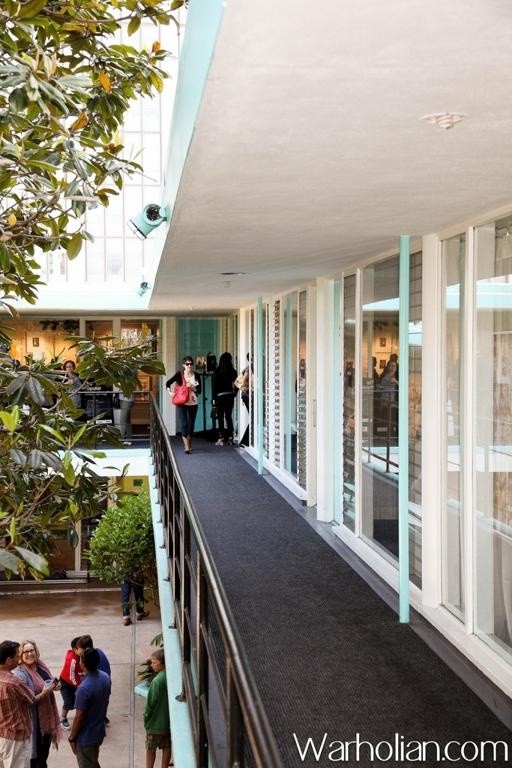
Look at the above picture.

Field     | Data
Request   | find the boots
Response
[182,435,192,453]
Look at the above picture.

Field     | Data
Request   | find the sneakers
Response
[60,717,71,731]
[137,610,149,620]
[124,618,132,626]
[215,438,245,447]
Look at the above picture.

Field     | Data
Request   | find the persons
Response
[76,634,112,725]
[59,637,87,730]
[120,539,151,626]
[16,352,253,454]
[367,353,397,443]
[68,648,113,768]
[142,648,172,768]
[1,640,37,768]
[11,638,62,768]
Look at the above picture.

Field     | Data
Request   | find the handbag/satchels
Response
[172,385,189,405]
[235,372,249,392]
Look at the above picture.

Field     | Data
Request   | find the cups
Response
[44,679,53,688]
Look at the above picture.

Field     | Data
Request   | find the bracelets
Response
[68,736,76,743]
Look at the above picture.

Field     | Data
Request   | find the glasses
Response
[186,363,193,366]
